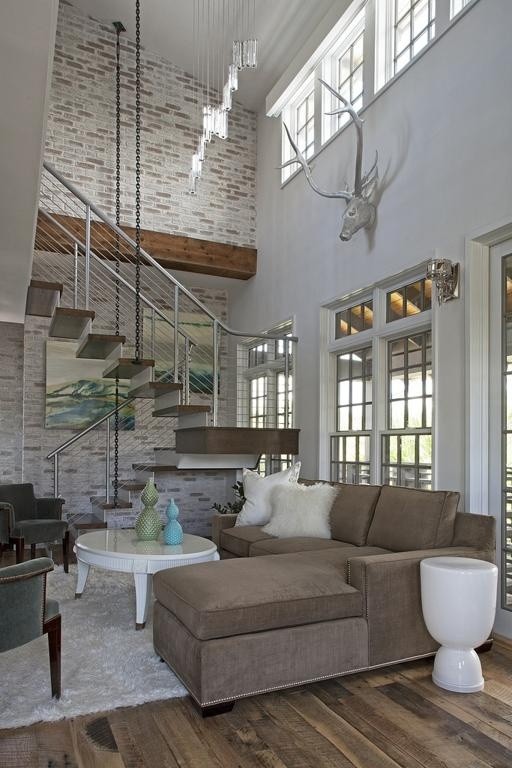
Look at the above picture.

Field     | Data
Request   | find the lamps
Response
[425,258,459,303]
[187,0,257,195]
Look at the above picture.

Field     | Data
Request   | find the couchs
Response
[151,514,498,719]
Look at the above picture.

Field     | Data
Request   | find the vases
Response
[163,498,184,545]
[134,477,163,541]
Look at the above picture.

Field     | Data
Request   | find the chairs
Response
[0,484,70,573]
[0,557,61,702]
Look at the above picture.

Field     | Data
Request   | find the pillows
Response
[233,451,460,553]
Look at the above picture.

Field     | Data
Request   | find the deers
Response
[273,77,378,241]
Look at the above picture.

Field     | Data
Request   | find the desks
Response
[418,557,497,693]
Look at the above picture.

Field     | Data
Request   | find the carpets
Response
[0,565,188,729]
